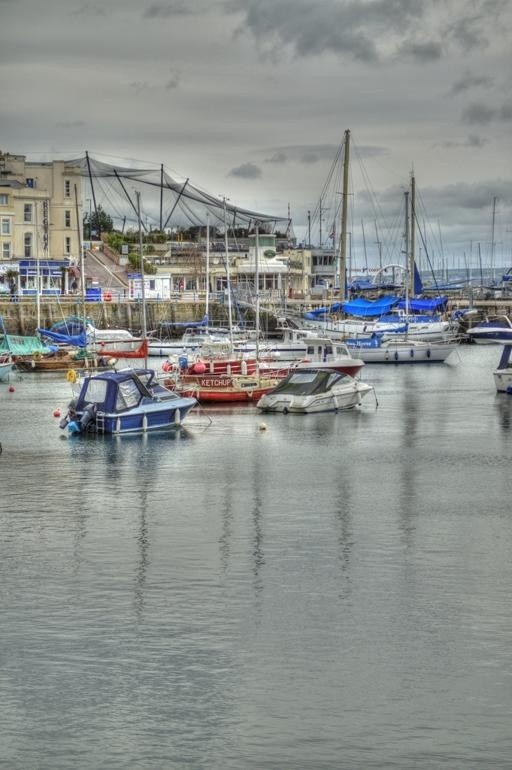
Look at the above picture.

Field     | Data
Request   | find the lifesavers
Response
[67,369,76,383]
[32,351,41,361]
[77,348,87,358]
[103,290,112,301]
